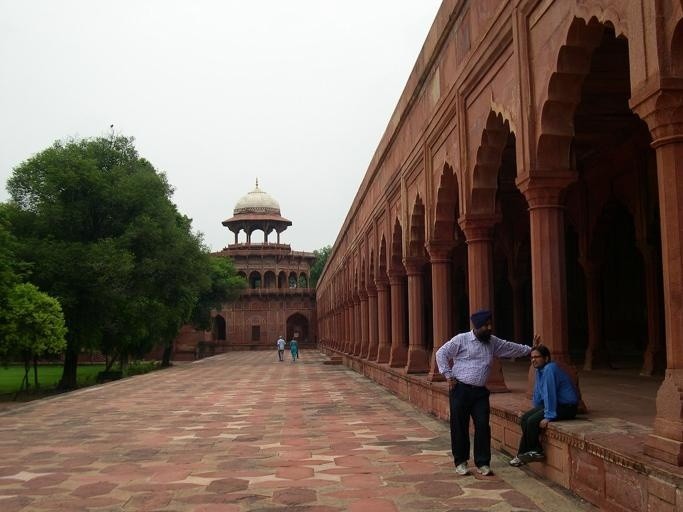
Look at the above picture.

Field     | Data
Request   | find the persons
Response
[289,336,298,361]
[508,345,583,468]
[276,335,285,361]
[434,309,543,477]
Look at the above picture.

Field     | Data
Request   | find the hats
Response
[470,310,493,329]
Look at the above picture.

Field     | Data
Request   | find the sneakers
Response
[456,460,470,475]
[509,448,545,467]
[477,464,490,475]
